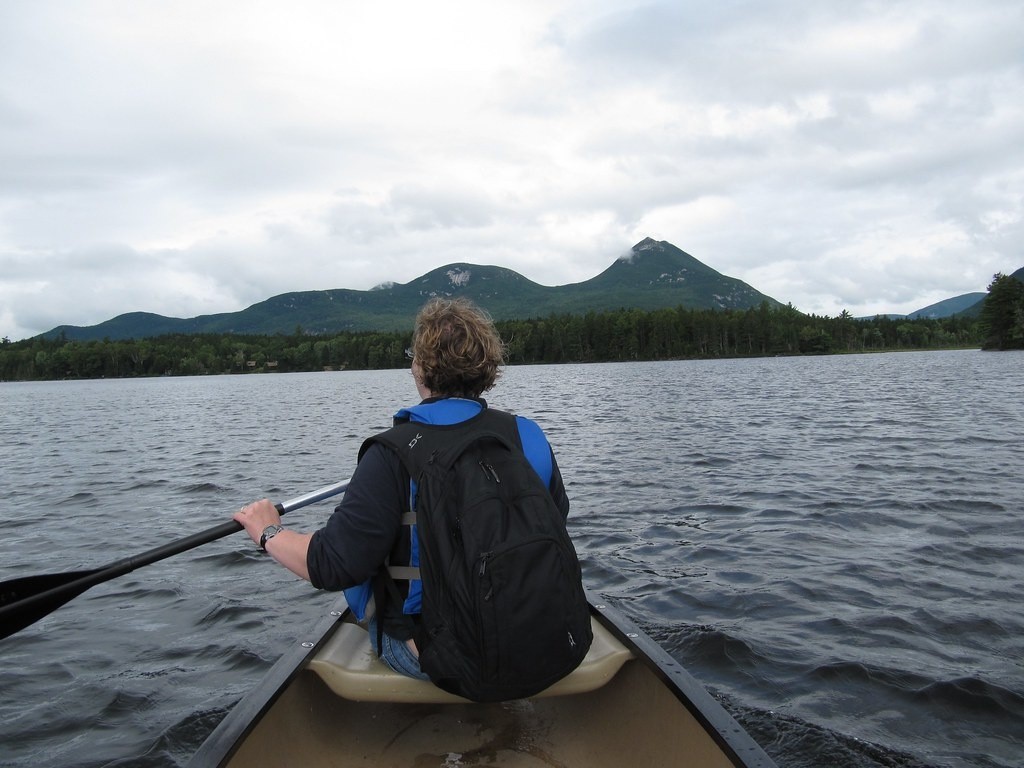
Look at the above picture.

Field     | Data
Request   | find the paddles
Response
[0,477,351,640]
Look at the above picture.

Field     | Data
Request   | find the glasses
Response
[404,346,417,359]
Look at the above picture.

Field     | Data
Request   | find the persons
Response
[232,298,571,681]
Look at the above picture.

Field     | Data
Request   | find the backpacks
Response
[362,406,596,705]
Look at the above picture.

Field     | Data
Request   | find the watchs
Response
[258,526,284,548]
